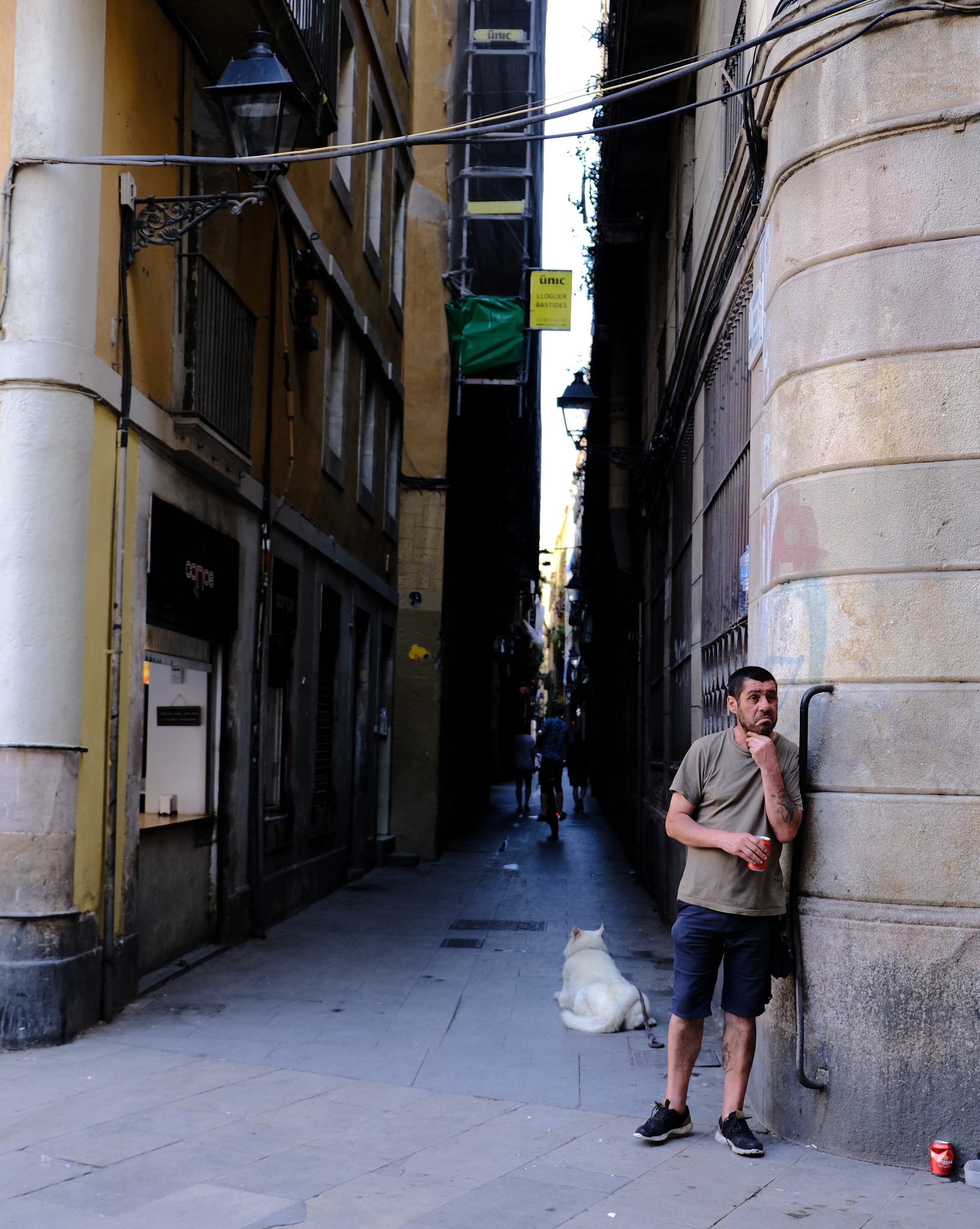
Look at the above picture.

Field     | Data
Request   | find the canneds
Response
[929,1139,953,1176]
[747,835,770,871]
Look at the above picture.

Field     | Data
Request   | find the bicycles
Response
[531,751,574,840]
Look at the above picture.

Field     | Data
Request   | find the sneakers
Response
[715,1109,764,1156]
[634,1097,693,1141]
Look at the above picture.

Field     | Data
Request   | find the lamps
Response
[111,20,310,275]
[554,370,645,470]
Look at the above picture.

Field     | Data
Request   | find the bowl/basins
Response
[963,1160,980,1187]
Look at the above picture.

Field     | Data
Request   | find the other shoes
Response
[524,805,530,812]
[559,812,567,821]
[573,799,584,811]
[537,813,548,822]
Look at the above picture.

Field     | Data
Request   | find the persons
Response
[633,665,804,1156]
[512,701,592,818]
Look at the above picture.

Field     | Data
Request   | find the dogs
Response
[553,921,656,1033]
